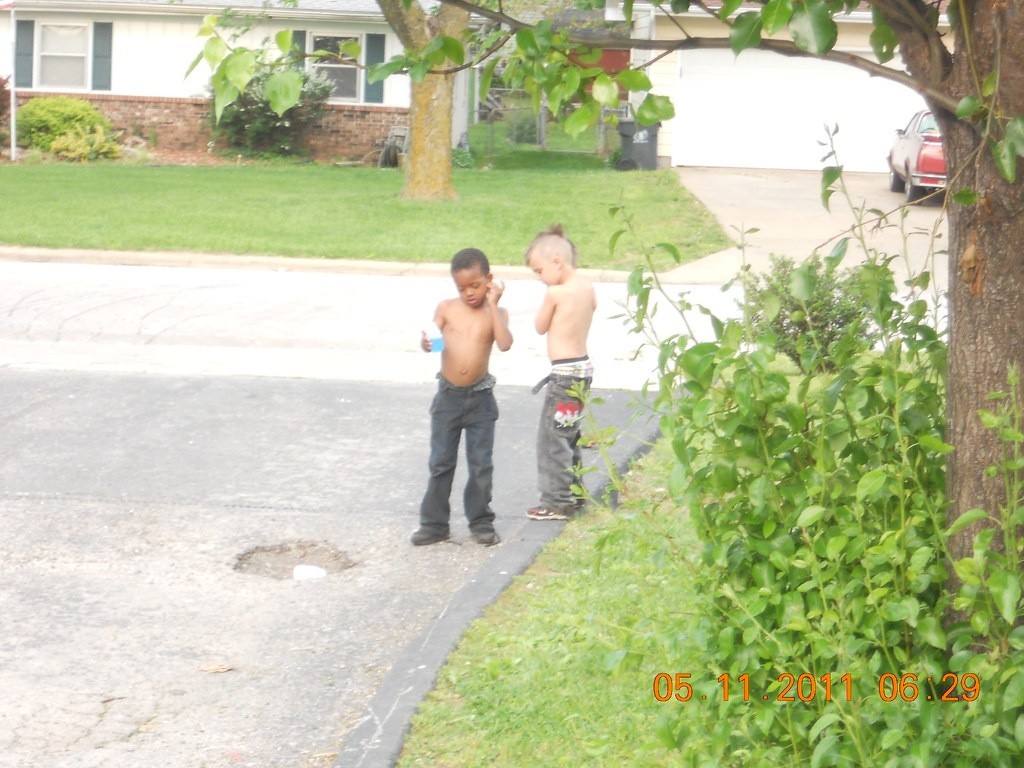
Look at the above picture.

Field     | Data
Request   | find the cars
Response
[886,109,947,203]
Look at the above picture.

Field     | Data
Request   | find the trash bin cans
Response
[616,117,662,171]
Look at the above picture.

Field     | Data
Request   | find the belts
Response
[532,376,592,395]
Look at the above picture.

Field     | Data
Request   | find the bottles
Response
[292,565,326,580]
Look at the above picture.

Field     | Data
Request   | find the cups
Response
[430,335,444,352]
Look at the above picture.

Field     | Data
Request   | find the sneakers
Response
[474,531,495,544]
[572,502,586,509]
[412,528,450,545]
[525,505,571,520]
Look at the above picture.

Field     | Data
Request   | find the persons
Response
[411,247,513,547]
[524,222,597,521]
